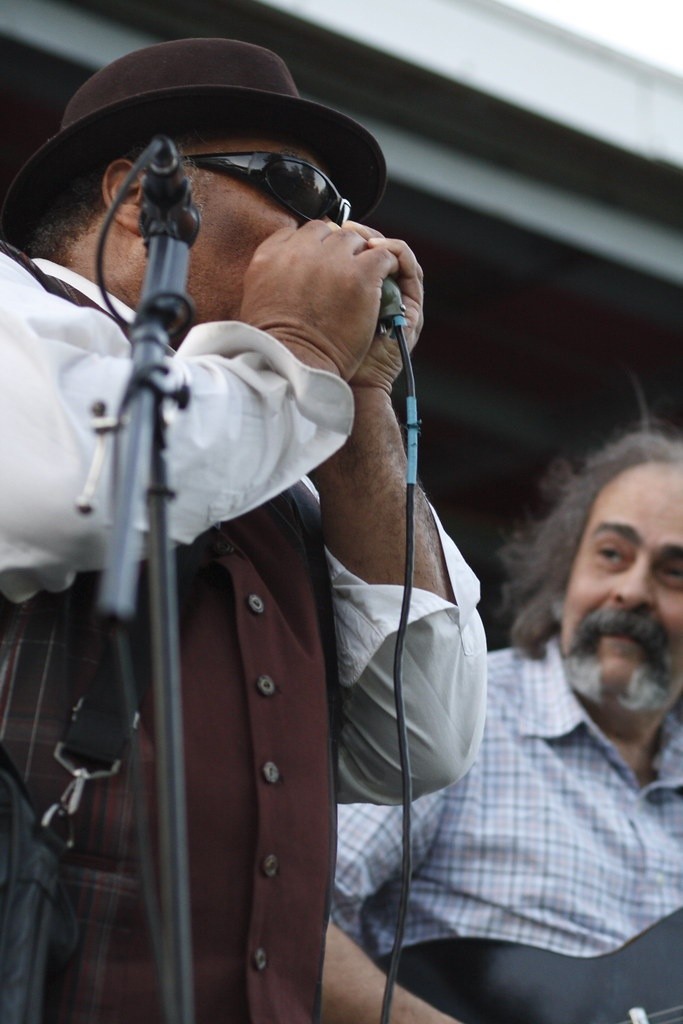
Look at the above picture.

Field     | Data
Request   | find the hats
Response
[0,36,385,240]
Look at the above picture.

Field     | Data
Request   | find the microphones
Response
[136,137,200,249]
[379,275,406,334]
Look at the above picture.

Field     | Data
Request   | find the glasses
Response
[183,150,352,233]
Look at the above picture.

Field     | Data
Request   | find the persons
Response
[0,37,491,1024]
[319,427,683,1024]
